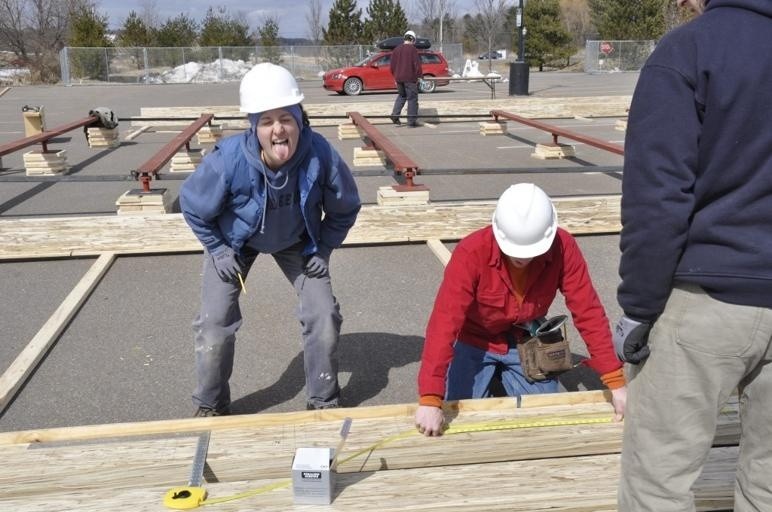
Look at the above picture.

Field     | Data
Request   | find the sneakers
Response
[390,114,401,124]
[195,407,229,417]
[306,400,343,410]
[407,123,417,127]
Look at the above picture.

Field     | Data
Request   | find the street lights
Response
[522,25,528,57]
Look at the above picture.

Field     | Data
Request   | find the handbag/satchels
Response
[517,334,574,383]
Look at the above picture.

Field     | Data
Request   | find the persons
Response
[390,30,424,127]
[614,0,772,512]
[179,61,362,418]
[414,183,628,437]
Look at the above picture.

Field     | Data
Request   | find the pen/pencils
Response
[238,273,246,294]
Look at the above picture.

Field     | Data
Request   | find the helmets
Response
[404,30,416,42]
[492,182,559,258]
[239,63,304,114]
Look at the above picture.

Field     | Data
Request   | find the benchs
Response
[421,76,502,99]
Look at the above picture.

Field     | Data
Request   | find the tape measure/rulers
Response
[187,428,211,488]
[165,416,624,507]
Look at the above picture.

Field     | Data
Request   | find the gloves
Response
[302,251,329,278]
[208,244,246,282]
[613,313,652,364]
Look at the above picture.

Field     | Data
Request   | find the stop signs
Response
[598,41,613,55]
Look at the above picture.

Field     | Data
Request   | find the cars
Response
[322,34,450,96]
[478,50,503,60]
